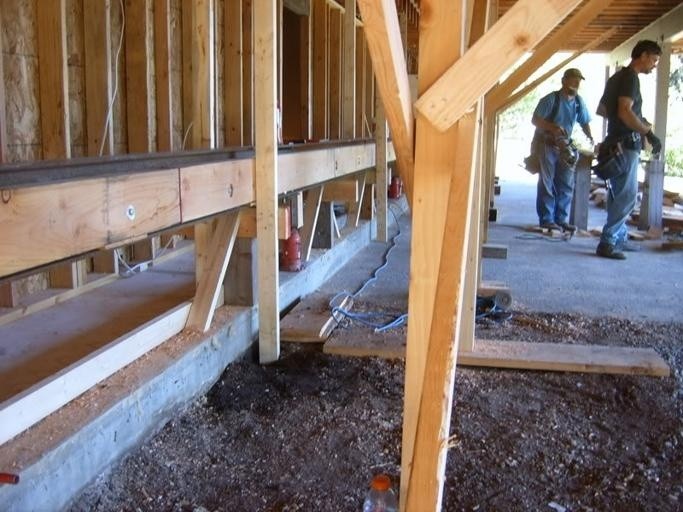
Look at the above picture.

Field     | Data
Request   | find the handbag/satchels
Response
[590,136,629,181]
[523,131,545,175]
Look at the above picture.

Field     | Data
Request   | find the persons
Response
[596,42,663,261]
[529,67,594,232]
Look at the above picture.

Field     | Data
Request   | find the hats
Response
[565,68,584,81]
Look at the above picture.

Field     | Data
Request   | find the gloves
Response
[644,128,662,155]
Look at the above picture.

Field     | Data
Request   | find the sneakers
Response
[596,242,626,259]
[557,222,578,232]
[616,240,641,252]
[540,222,561,231]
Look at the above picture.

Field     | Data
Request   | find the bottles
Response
[391,176,400,200]
[279,228,302,272]
[362,476,396,511]
